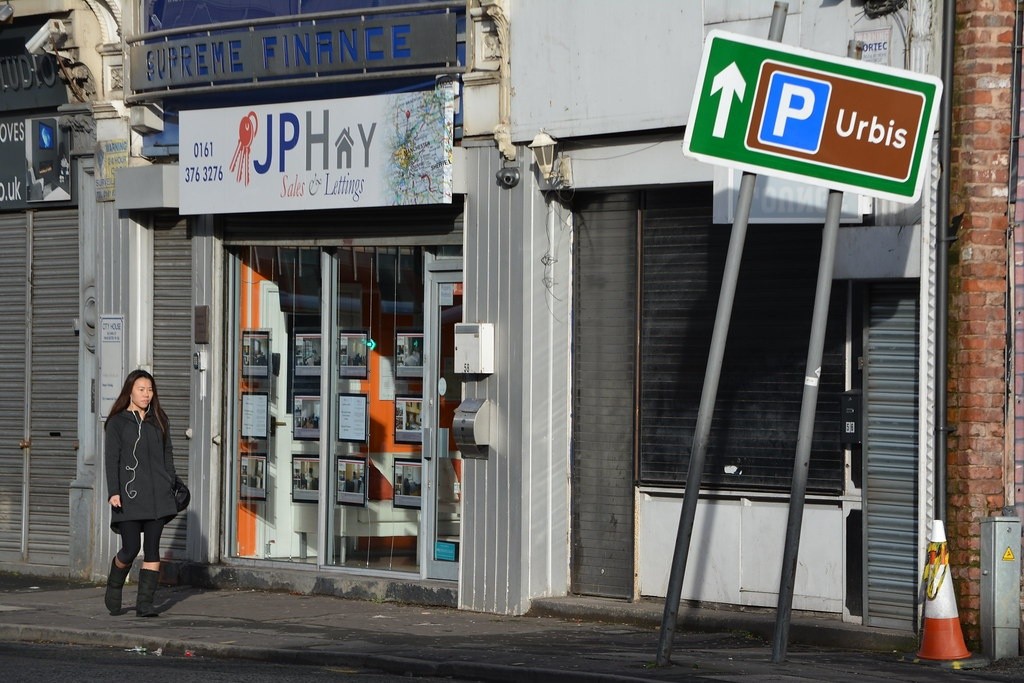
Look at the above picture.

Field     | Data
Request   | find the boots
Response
[136,568,160,617]
[105,556,132,613]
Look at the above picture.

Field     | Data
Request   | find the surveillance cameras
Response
[25,19,66,55]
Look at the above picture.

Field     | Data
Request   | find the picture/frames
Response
[336,455,367,508]
[338,392,367,444]
[241,391,268,441]
[242,331,270,379]
[393,457,422,511]
[240,453,267,501]
[291,454,319,504]
[294,331,321,377]
[394,394,422,446]
[339,330,370,380]
[395,329,424,380]
[293,392,320,441]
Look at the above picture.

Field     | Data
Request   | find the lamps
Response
[527,128,572,186]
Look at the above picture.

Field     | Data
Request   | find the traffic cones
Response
[917,542,972,659]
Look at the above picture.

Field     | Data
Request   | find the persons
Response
[103,369,178,619]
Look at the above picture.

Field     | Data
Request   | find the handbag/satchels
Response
[174,476,190,512]
